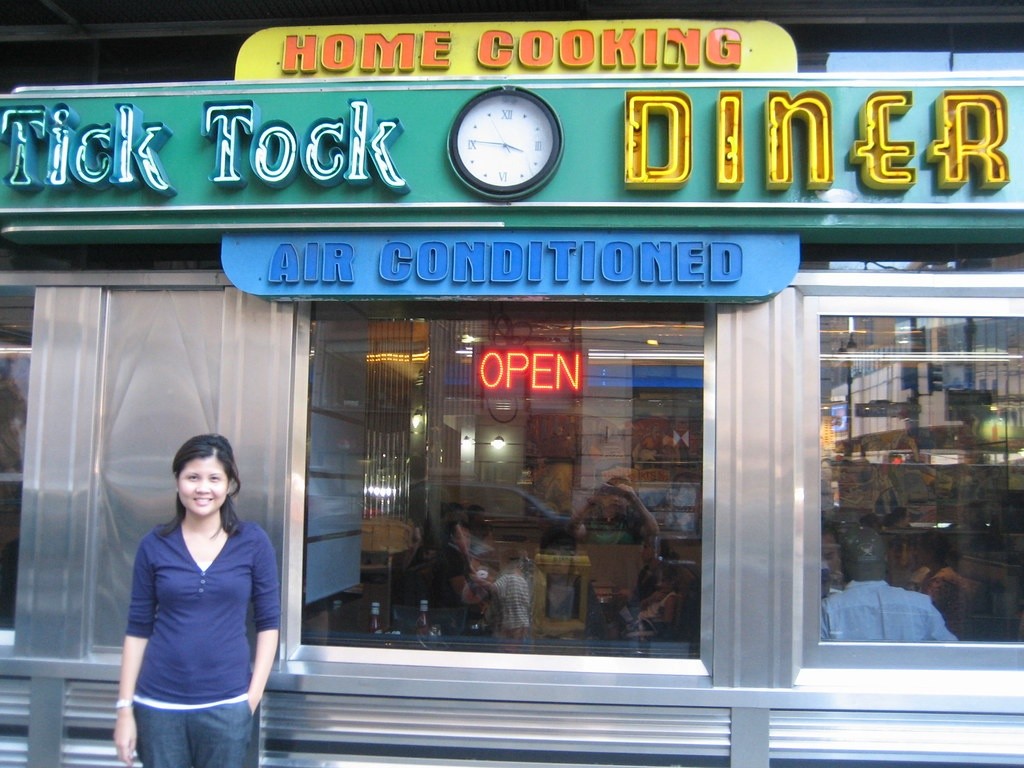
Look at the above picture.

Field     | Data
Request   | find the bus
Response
[835,424,991,504]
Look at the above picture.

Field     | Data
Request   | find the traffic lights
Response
[900,362,914,389]
[928,364,944,396]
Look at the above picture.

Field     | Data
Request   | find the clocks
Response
[445,82,567,203]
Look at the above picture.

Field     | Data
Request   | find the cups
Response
[429,624,441,636]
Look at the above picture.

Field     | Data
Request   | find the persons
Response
[913,534,1004,623]
[823,530,957,642]
[112,434,281,768]
[818,433,1013,597]
[379,455,702,649]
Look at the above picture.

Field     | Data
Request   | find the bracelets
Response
[115,699,133,709]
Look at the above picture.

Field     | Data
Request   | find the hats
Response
[540,525,576,549]
[842,527,886,581]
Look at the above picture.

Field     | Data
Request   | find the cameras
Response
[606,485,625,496]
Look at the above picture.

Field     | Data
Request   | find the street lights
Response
[836,335,859,457]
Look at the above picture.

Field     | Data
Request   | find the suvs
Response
[393,479,582,554]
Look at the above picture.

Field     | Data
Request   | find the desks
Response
[881,527,996,567]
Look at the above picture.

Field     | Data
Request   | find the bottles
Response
[367,602,383,634]
[415,600,431,636]
[328,601,345,634]
